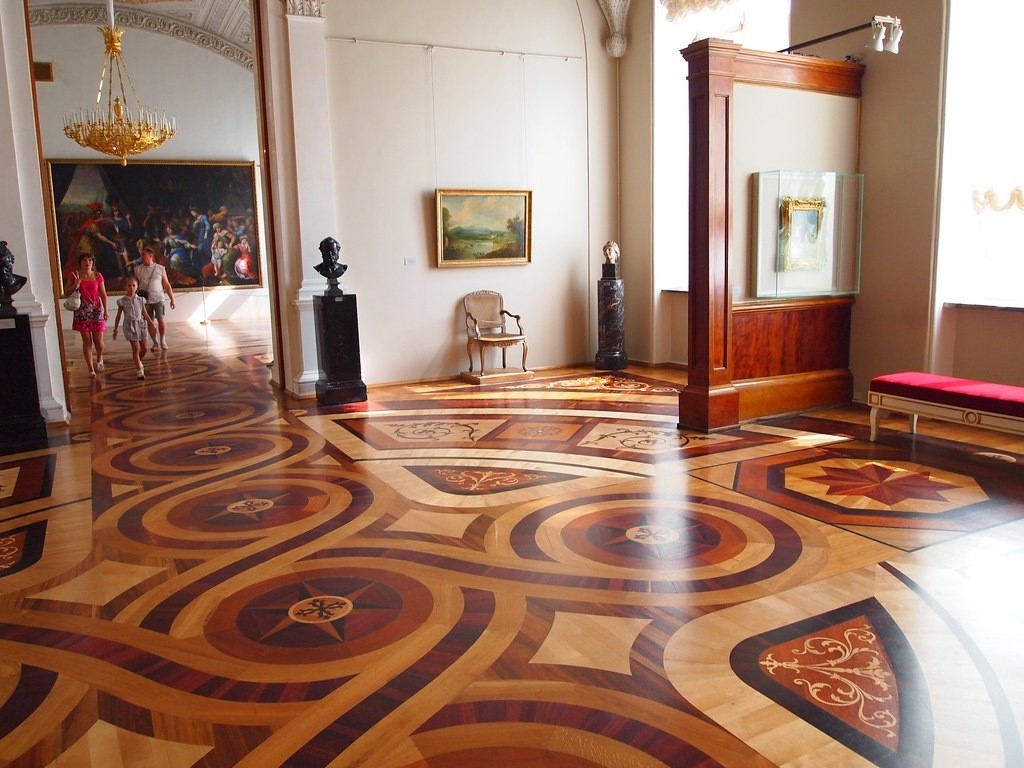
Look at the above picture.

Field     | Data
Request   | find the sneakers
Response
[136,361,145,380]
[151,341,169,352]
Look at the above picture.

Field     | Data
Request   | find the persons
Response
[313,237,348,279]
[64,252,110,378]
[132,248,175,352]
[112,278,157,381]
[602,240,621,263]
[0,241,28,296]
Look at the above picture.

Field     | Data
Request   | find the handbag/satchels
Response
[137,290,149,300]
[63,270,82,311]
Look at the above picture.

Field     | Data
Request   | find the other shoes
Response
[88,359,105,378]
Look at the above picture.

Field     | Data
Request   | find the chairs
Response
[462,289,529,374]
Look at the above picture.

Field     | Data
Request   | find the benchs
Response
[868,371,1024,444]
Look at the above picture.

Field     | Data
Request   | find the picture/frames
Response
[45,158,261,298]
[434,188,534,268]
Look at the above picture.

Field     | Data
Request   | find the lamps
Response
[63,0,178,166]
[866,14,905,53]
